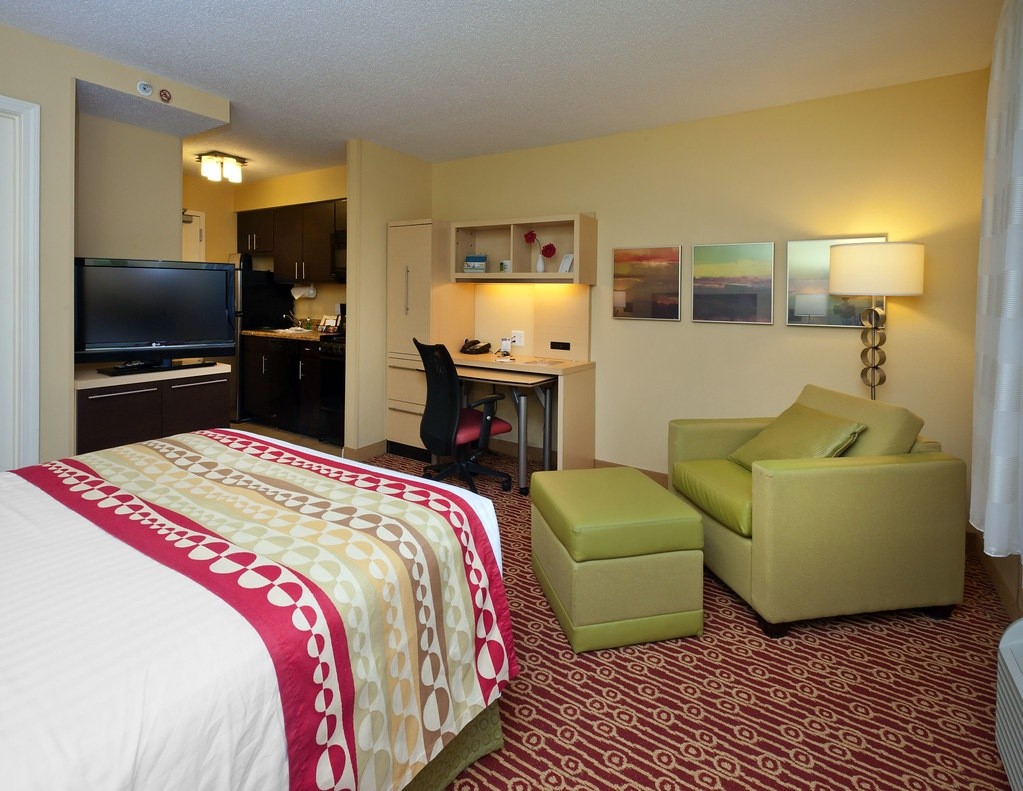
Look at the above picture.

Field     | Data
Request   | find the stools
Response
[529,466,704,653]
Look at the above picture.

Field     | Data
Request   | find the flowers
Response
[524,230,557,259]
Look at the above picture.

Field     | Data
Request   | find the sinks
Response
[263,328,313,333]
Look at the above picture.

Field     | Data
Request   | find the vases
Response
[536,254,545,273]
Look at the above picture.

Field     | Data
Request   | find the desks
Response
[416,366,557,496]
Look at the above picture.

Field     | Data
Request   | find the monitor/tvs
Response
[74,257,236,377]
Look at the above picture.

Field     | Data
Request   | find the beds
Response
[0,421,522,791]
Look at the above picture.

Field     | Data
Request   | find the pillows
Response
[726,404,866,478]
[794,382,925,461]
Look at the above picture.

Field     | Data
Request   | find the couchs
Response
[668,418,968,636]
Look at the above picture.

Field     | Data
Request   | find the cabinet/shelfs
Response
[385,214,598,472]
[76,372,264,457]
[235,198,347,448]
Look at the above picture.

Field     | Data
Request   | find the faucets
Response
[283,310,302,328]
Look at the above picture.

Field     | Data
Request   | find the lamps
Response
[196,151,248,186]
[826,241,926,402]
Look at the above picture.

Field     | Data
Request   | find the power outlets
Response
[510,330,524,347]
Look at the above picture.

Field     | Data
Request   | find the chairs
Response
[411,338,513,497]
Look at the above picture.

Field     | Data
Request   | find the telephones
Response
[459,339,491,355]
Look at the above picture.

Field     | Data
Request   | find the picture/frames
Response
[785,237,886,331]
[558,253,574,272]
[611,246,682,322]
[690,240,776,326]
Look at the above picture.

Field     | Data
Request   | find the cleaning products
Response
[306,316,311,329]
